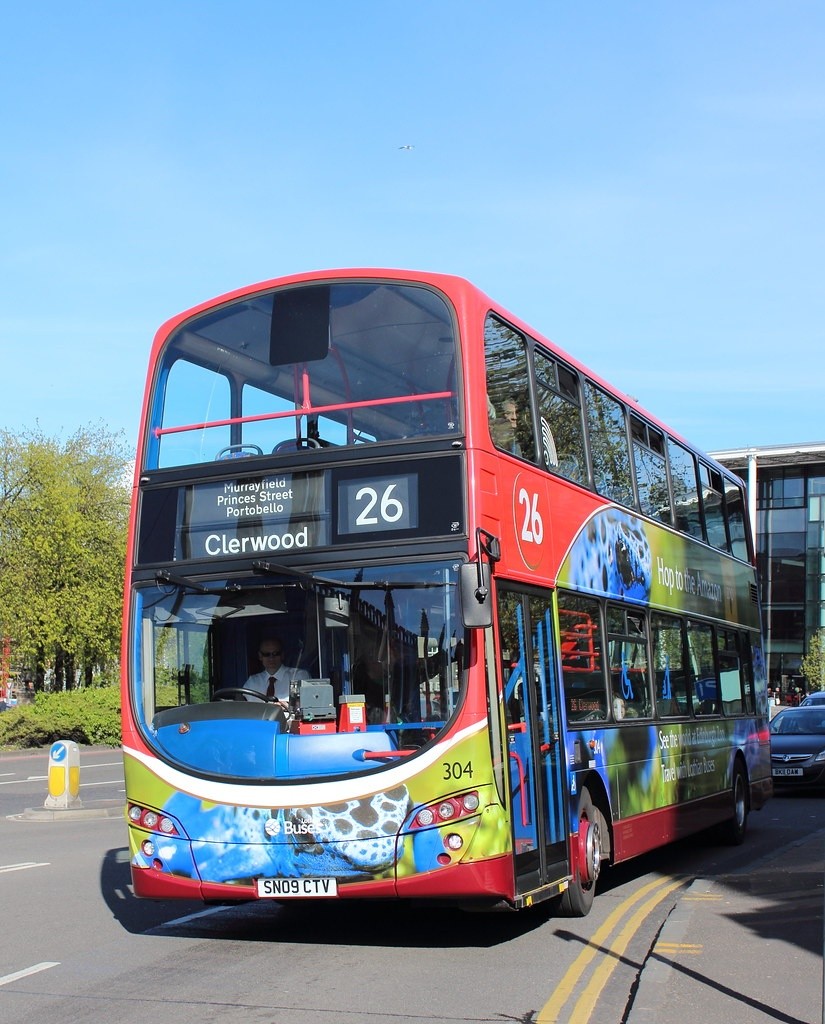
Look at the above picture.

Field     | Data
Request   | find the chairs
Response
[218,438,322,462]
[558,456,632,507]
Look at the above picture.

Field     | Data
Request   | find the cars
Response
[768,704,825,793]
[794,692,825,716]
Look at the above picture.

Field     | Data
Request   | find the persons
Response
[486,393,496,420]
[241,638,313,733]
[501,396,536,463]
[789,679,799,707]
[507,631,680,843]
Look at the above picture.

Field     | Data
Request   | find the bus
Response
[117,265,776,920]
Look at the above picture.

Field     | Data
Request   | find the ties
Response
[266,677,276,698]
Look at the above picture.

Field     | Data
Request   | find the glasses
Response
[259,649,282,657]
